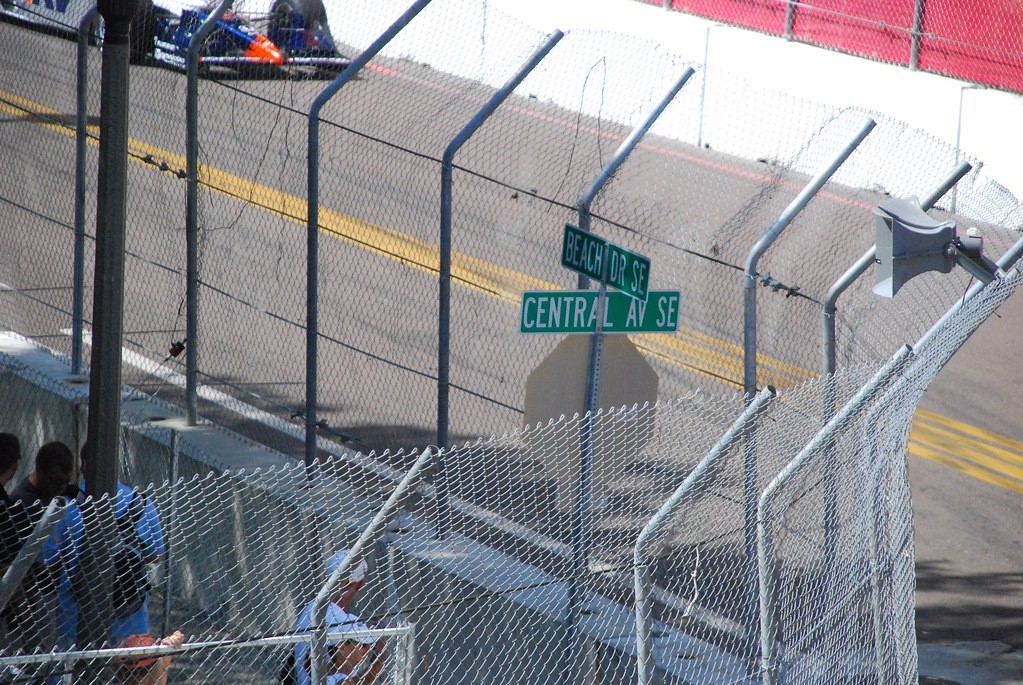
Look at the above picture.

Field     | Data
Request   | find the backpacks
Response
[76,488,148,619]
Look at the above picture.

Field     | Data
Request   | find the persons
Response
[294,549,390,685]
[0,432,184,685]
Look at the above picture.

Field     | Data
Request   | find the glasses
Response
[40,463,74,487]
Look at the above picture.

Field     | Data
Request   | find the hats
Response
[117,633,159,669]
[326,549,368,587]
[327,614,378,647]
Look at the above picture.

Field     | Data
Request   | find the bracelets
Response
[371,652,386,666]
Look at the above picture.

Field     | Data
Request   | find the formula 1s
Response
[0,0,356,76]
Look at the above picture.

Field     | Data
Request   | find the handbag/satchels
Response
[278,643,297,685]
